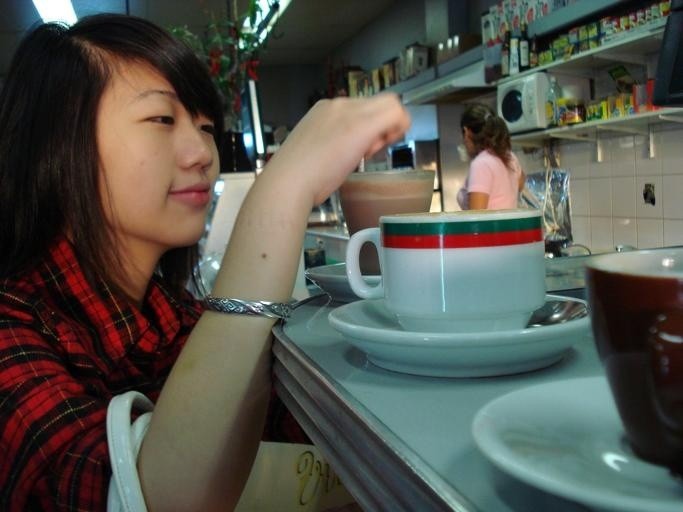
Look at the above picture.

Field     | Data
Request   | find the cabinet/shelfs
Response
[499,25,683,162]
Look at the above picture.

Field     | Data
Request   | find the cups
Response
[344,210,550,334]
[337,169,436,276]
[582,246,683,470]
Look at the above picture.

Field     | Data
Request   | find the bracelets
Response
[201,296,289,322]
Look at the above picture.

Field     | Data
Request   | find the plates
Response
[327,294,592,380]
[468,376,682,512]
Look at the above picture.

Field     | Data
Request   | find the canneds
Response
[565,98,585,125]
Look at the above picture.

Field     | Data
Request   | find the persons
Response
[455,103,525,211]
[0,13,412,511]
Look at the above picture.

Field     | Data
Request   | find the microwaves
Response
[492,71,593,139]
